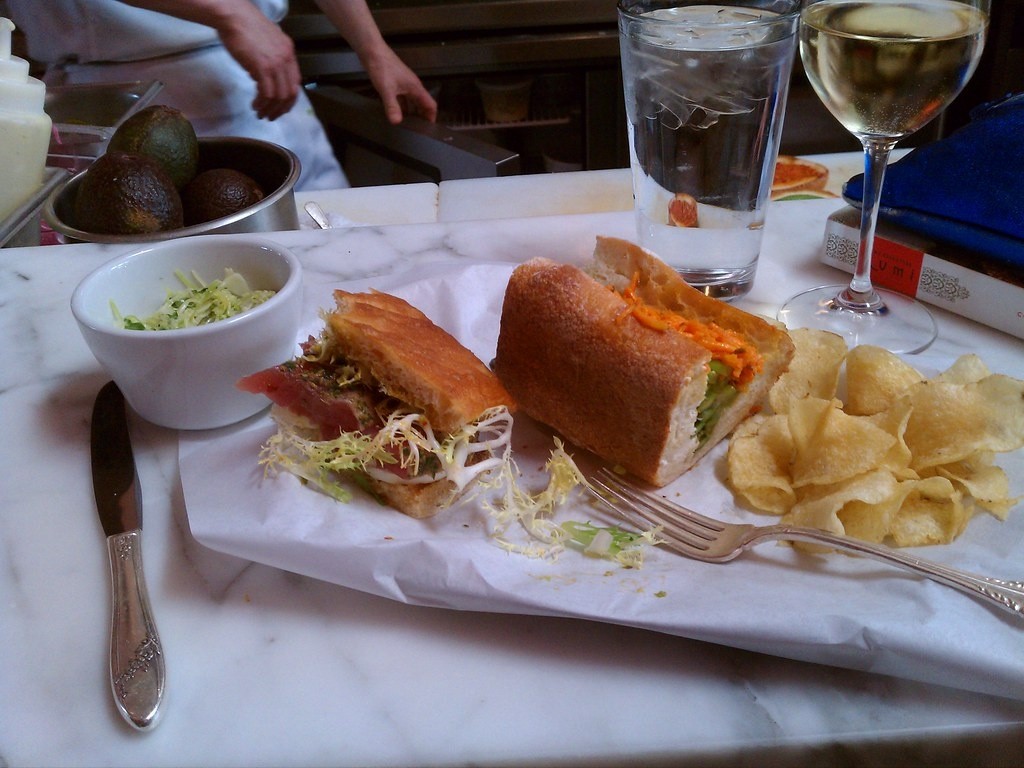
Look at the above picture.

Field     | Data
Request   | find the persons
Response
[8,0,436,193]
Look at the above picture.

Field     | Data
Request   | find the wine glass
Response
[776,0,992,356]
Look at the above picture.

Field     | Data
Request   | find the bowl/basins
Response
[474,73,533,123]
[42,136,302,243]
[70,234,304,431]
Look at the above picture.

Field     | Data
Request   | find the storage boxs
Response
[820,204,1024,340]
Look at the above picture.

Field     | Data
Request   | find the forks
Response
[585,466,1024,620]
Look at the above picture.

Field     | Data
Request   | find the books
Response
[821,206,1024,343]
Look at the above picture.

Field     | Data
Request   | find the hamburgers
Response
[235,286,669,571]
[491,234,795,486]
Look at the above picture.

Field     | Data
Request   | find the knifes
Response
[89,380,168,731]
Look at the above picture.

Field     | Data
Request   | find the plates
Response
[768,154,841,201]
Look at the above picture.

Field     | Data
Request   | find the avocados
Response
[71,104,265,235]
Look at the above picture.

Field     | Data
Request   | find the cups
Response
[617,0,803,302]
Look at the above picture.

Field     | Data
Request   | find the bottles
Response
[0,18,53,225]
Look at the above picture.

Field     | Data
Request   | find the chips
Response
[728,327,1024,558]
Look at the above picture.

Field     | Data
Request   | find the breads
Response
[773,154,841,200]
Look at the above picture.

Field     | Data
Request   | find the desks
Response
[0,194,1024,768]
